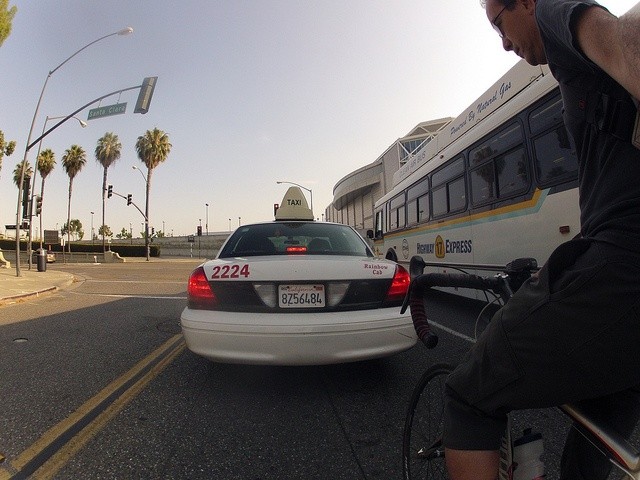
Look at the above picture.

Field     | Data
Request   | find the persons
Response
[445,0,640,480]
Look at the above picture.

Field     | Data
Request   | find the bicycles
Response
[400,258,640,479]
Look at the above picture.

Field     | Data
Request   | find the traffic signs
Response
[87,102,126,120]
[188,235,194,242]
[197,226,202,236]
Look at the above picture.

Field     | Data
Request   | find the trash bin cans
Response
[36,248,47,271]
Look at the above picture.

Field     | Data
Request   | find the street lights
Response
[277,181,312,209]
[239,217,240,226]
[199,219,201,226]
[17,27,132,275]
[91,211,94,239]
[206,203,208,235]
[132,166,146,182]
[229,219,231,231]
[141,223,144,232]
[29,116,87,268]
[163,221,164,234]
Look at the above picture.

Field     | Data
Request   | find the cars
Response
[28,249,55,263]
[181,186,418,365]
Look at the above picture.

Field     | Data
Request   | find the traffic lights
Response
[23,178,31,206]
[108,185,112,198]
[35,194,42,217]
[152,227,154,234]
[127,194,132,205]
[134,77,158,114]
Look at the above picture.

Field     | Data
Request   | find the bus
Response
[367,59,581,306]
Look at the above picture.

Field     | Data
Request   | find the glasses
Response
[491,6,508,37]
